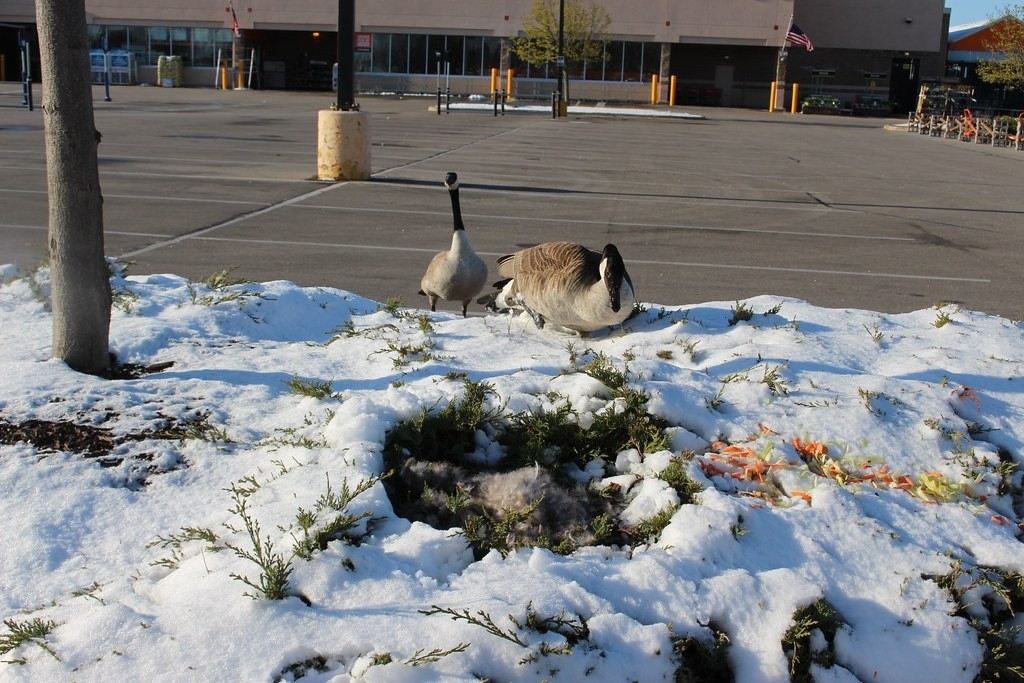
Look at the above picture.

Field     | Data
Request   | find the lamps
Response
[904,17,913,23]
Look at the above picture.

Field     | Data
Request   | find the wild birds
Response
[495,241,635,337]
[418,171,488,318]
[476,277,543,330]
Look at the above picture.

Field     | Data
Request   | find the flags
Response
[786,24,814,51]
